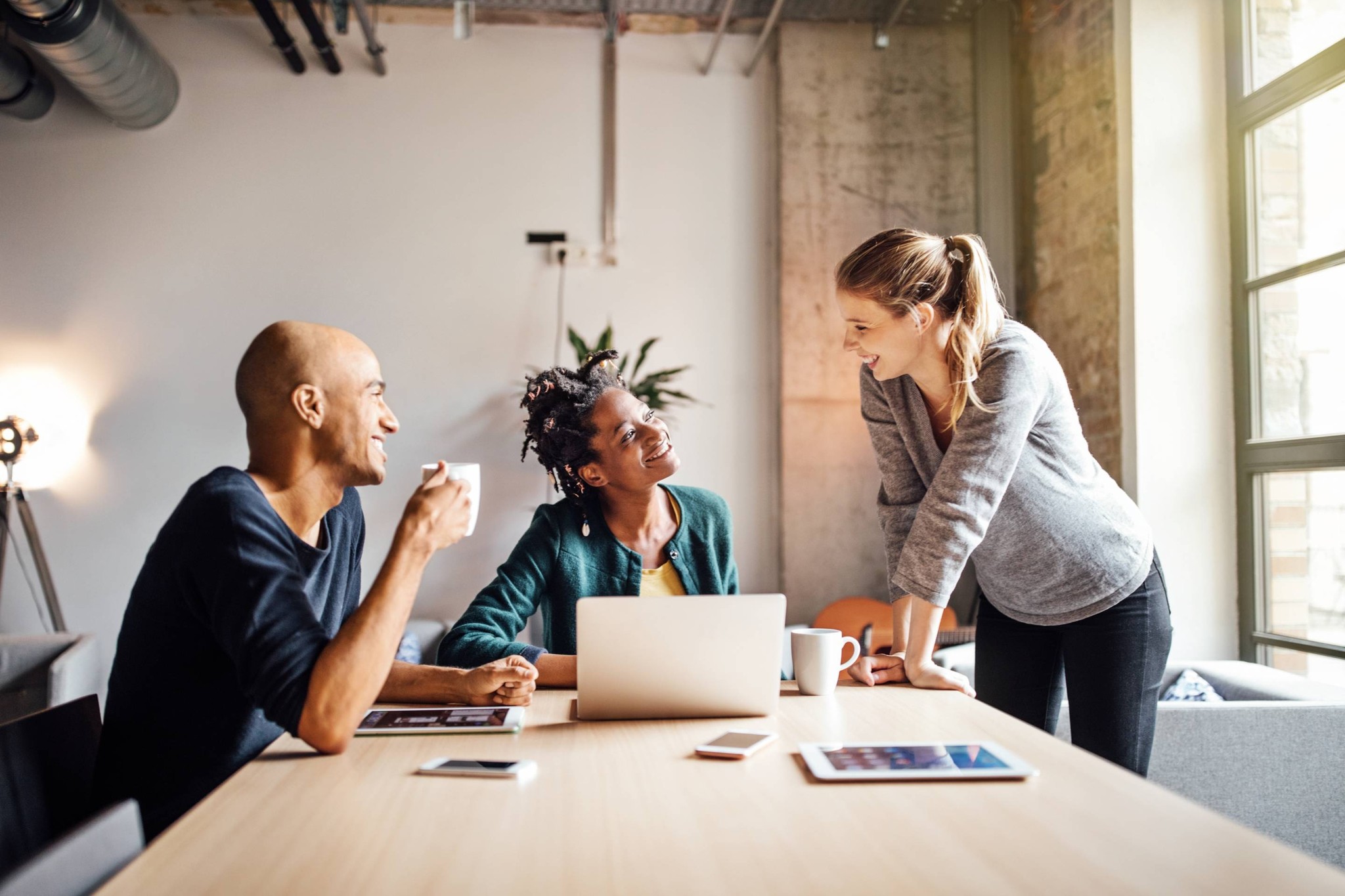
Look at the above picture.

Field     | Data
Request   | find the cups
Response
[423,463,480,537]
[791,628,861,695]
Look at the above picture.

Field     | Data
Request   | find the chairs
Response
[0,632,145,896]
[935,641,1344,870]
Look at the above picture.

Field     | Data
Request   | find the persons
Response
[435,348,740,686]
[834,227,1174,779]
[89,320,539,843]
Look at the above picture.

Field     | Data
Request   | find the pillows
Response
[1160,667,1223,702]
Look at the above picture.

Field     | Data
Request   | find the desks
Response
[88,679,1345,896]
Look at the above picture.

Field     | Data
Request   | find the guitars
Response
[812,597,976,680]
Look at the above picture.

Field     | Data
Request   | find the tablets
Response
[353,705,525,735]
[798,741,1040,781]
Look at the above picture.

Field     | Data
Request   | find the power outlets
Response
[550,240,572,264]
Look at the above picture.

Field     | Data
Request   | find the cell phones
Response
[695,730,778,759]
[418,758,534,777]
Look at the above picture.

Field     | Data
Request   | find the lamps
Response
[0,414,67,632]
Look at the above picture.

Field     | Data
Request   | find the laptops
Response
[576,594,787,719]
